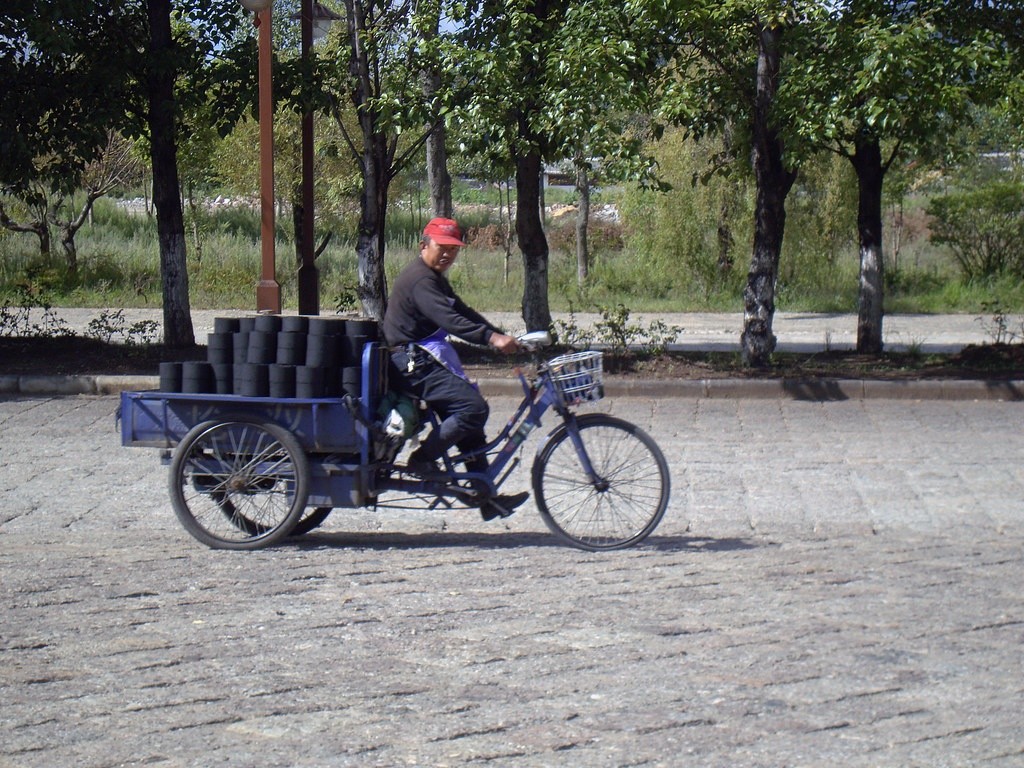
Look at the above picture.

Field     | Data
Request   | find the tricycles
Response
[115,329,672,551]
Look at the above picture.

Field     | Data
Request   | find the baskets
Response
[547,350,604,405]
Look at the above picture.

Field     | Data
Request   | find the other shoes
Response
[408,454,454,480]
[481,491,529,520]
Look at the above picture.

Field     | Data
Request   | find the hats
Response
[424,217,465,246]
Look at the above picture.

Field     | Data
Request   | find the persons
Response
[382,218,531,522]
